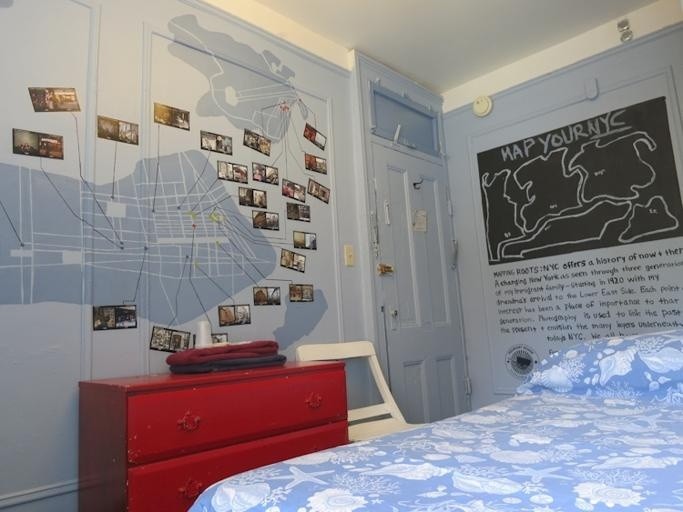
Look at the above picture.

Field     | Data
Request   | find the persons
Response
[253,166,261,180]
[44,89,58,110]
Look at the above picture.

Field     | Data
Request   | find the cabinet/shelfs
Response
[78,361,349,512]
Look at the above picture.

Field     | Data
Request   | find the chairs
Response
[294,340,431,443]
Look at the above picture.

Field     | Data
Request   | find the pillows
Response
[517,329,683,398]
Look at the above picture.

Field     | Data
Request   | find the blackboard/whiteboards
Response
[477,96,683,265]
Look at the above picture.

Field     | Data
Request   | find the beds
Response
[184,396,683,512]
